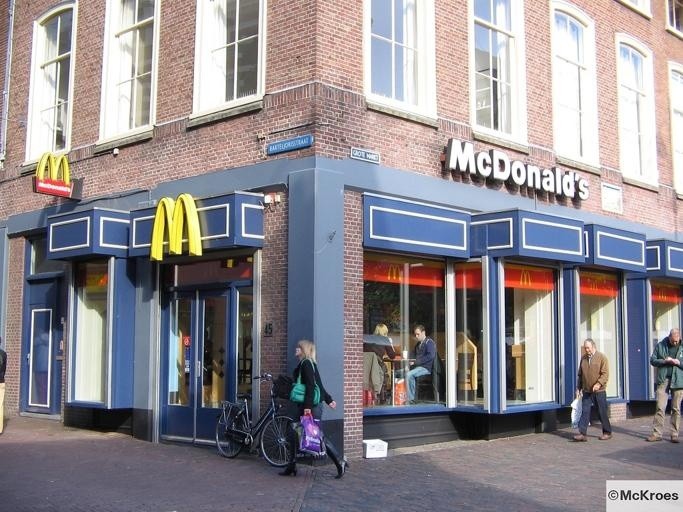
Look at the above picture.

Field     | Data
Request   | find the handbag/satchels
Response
[570,395,591,429]
[297,413,326,455]
[289,383,321,406]
[274,375,292,400]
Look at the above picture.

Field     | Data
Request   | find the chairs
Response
[416,350,446,403]
[363,351,388,408]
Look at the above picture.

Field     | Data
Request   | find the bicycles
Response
[215,372,295,468]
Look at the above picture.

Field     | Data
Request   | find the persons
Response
[571,337,614,442]
[0,336,9,435]
[363,323,397,406]
[392,323,438,403]
[645,327,682,445]
[277,340,350,480]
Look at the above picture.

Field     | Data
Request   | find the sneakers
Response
[599,433,612,440]
[671,435,680,443]
[573,434,587,442]
[647,434,662,442]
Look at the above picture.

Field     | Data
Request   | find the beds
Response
[383,359,416,406]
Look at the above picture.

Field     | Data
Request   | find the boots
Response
[278,434,298,476]
[322,435,349,479]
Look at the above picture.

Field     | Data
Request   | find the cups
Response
[402,351,408,359]
